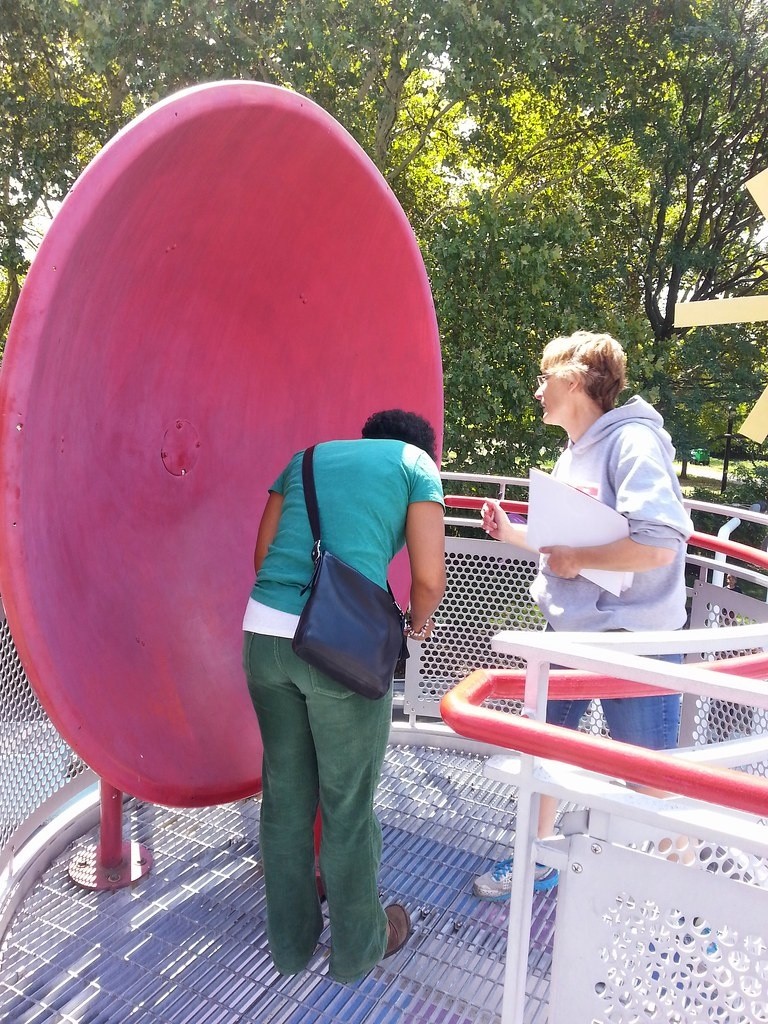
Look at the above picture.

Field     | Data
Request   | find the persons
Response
[242,409,447,983]
[723,574,743,627]
[473,332,719,955]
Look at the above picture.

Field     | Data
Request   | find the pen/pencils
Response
[485,491,503,540]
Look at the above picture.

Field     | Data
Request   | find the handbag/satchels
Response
[292,550,410,700]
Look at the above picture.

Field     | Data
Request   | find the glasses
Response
[536,372,556,386]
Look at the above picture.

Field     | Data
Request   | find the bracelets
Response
[403,603,428,638]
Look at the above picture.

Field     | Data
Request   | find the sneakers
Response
[473,857,559,900]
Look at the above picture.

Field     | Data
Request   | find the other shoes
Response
[362,904,411,961]
